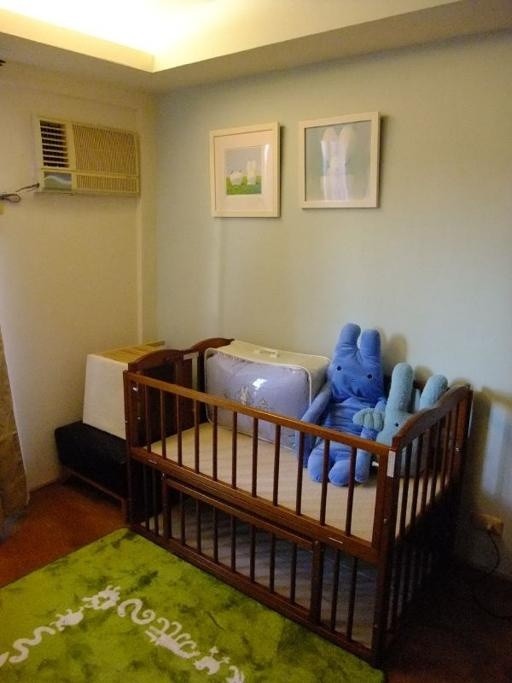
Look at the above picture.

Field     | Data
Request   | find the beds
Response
[121,337,473,669]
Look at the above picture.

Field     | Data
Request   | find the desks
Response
[54,421,169,524]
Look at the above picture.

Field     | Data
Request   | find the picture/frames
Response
[297,111,380,209]
[209,121,280,219]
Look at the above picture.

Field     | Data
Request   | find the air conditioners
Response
[31,113,140,199]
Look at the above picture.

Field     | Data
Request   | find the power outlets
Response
[474,511,504,537]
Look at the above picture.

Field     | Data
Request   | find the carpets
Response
[0,528,382,683]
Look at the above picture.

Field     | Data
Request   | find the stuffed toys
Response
[300,322,447,487]
